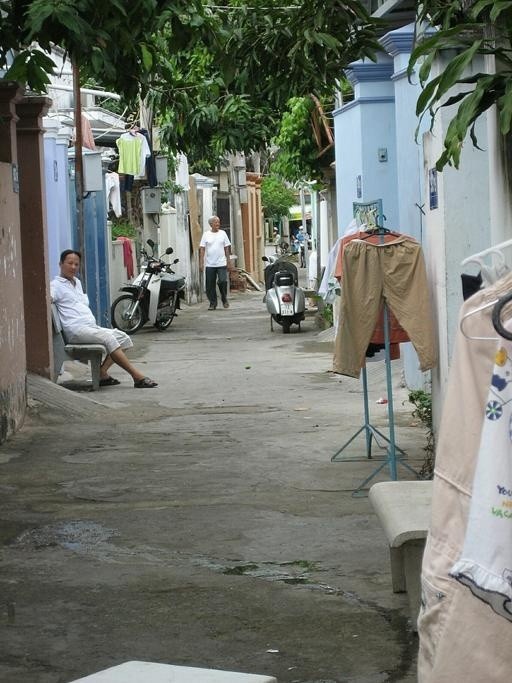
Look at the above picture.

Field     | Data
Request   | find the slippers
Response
[100,376,121,386]
[132,376,160,388]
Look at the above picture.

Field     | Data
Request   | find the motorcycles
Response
[111,236,186,333]
[257,249,307,333]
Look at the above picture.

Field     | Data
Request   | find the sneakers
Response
[207,301,229,310]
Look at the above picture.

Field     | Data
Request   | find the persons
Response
[48,249,158,387]
[198,215,234,311]
[273,226,281,256]
[291,223,301,245]
[296,225,309,268]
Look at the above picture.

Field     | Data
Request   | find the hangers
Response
[353,201,399,243]
[461,230,510,344]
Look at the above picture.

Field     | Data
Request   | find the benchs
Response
[51,303,106,390]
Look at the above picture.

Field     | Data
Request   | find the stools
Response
[369,477,435,635]
